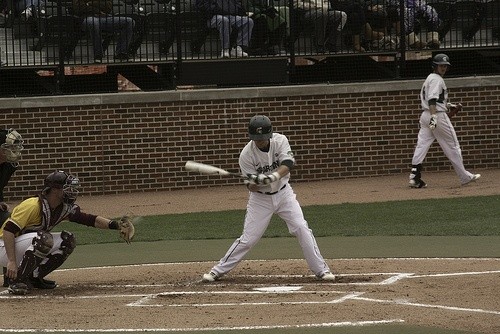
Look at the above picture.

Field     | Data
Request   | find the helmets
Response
[44,170,74,189]
[0,129,21,144]
[248,115,274,141]
[432,54,451,66]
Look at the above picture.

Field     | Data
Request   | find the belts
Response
[257,184,286,195]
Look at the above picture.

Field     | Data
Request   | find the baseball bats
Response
[447,103,463,118]
[185,160,255,181]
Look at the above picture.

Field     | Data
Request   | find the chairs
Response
[0,0,304,48]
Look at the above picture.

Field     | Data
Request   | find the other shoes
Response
[409,179,427,188]
[316,271,335,281]
[461,173,480,186]
[203,272,218,282]
[221,48,229,58]
[3,267,57,289]
[230,46,248,58]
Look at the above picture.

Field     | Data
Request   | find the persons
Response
[0,128,24,286]
[0,0,487,62]
[408,54,481,188]
[202,115,335,283]
[0,171,135,294]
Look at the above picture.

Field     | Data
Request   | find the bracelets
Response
[272,172,281,181]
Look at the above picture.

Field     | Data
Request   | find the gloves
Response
[246,172,281,193]
[447,103,462,114]
[428,115,437,128]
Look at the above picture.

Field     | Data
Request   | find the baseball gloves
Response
[118,218,136,243]
